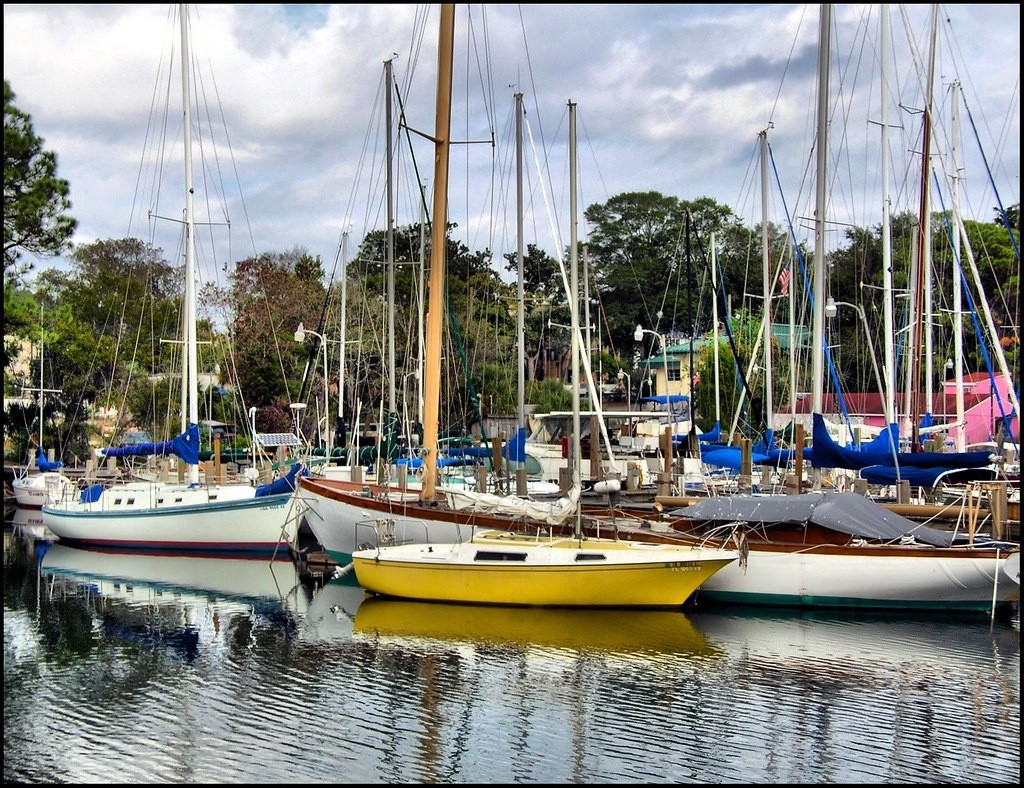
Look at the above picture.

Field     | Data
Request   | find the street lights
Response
[825,297,900,504]
[293,324,330,468]
[401,370,421,434]
[248,407,256,472]
[633,325,672,427]
[639,378,652,402]
[615,366,632,414]
[289,403,306,458]
[943,357,954,442]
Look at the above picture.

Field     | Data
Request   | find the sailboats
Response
[2,1,1021,618]
